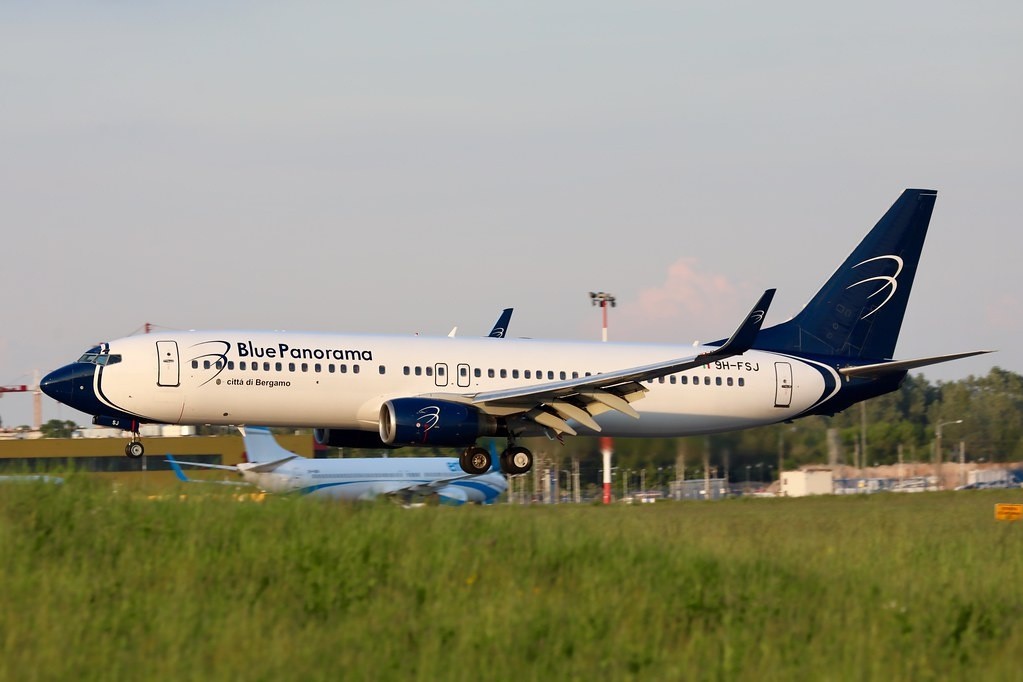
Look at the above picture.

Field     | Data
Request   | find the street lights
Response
[935,420,964,479]
[586,288,617,506]
[778,428,796,472]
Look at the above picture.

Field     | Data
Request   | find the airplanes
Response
[161,425,510,510]
[37,186,997,478]
